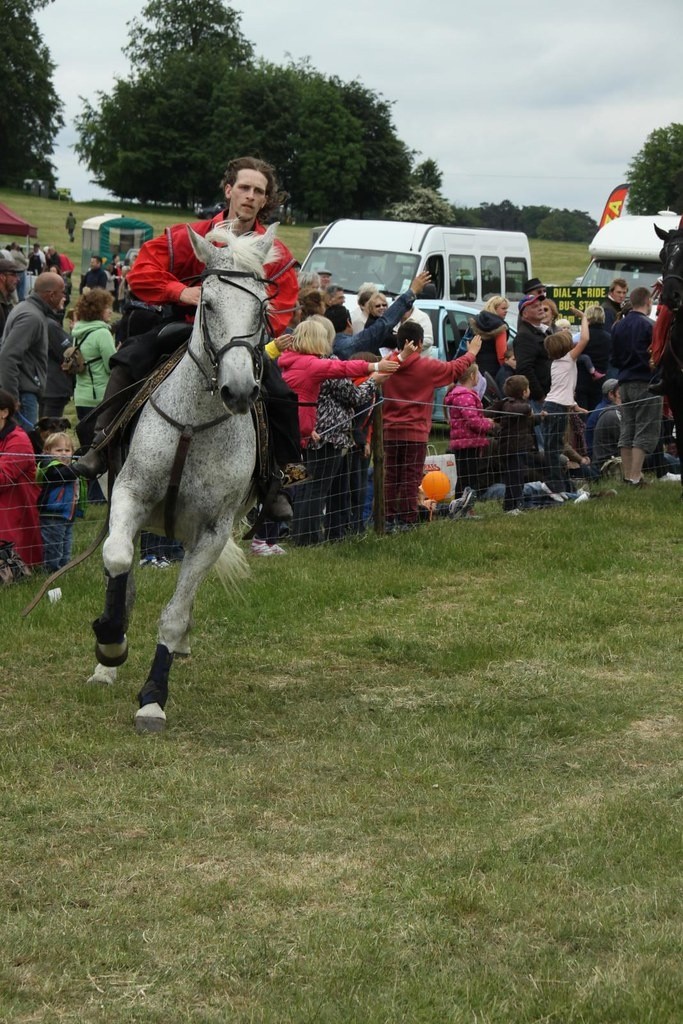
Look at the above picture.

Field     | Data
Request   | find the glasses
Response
[537,289,547,294]
[375,304,388,307]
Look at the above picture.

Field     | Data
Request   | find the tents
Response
[0,202,38,256]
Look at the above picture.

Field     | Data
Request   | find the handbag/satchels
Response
[0,540,31,585]
[423,445,458,506]
[453,327,474,359]
[522,482,550,508]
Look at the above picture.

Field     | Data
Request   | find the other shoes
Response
[649,378,666,394]
[449,487,477,521]
[541,483,563,502]
[560,492,568,500]
[507,508,526,516]
[595,372,606,380]
[624,479,652,489]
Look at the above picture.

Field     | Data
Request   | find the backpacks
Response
[61,330,100,374]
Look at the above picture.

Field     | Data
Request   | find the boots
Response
[73,364,138,478]
[271,494,293,518]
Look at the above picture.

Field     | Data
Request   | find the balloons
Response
[422,471,451,500]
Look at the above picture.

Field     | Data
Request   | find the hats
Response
[318,270,331,276]
[518,295,545,313]
[523,278,545,294]
[0,249,25,271]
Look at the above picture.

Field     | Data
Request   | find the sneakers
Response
[271,544,286,554]
[140,556,173,573]
[252,534,272,556]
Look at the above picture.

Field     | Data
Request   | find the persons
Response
[75,157,303,522]
[0,243,138,573]
[250,269,680,555]
[66,212,77,243]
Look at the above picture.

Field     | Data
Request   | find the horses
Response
[89,218,280,735]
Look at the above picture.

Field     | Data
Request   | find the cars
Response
[389,300,517,422]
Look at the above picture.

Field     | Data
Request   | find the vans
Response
[571,210,681,331]
[294,219,532,337]
